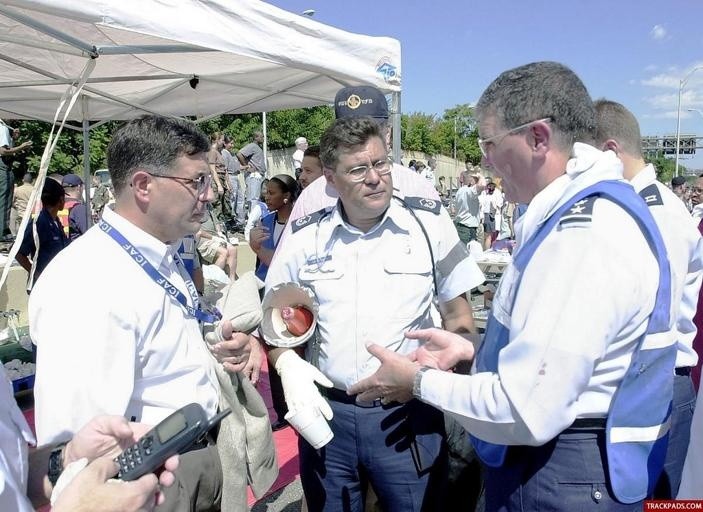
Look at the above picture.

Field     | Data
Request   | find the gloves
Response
[275,348,335,425]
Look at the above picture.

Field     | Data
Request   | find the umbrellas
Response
[0,0,403,230]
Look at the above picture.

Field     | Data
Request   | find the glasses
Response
[475,118,554,161]
[691,187,702,192]
[130,169,215,192]
[334,156,392,182]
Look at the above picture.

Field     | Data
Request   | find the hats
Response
[334,86,389,119]
[23,173,32,181]
[44,175,68,197]
[671,175,685,185]
[61,173,88,190]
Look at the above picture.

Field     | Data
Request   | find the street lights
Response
[455,106,476,174]
[688,109,703,116]
[676,66,703,177]
[262,10,317,180]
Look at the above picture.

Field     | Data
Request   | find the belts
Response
[217,171,228,176]
[229,173,239,174]
[320,384,403,409]
[456,223,479,229]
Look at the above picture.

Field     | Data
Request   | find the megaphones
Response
[257,282,320,348]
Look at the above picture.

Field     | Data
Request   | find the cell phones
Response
[97,402,232,481]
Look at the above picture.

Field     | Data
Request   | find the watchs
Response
[412,366,436,403]
[49,438,72,487]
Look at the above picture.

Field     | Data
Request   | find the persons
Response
[207,132,238,228]
[690,174,703,237]
[505,200,518,237]
[408,160,417,173]
[0,118,33,251]
[259,114,486,512]
[481,177,501,248]
[473,166,485,184]
[591,99,703,501]
[26,113,252,512]
[415,161,424,175]
[192,208,237,281]
[420,158,437,187]
[235,131,266,213]
[434,176,448,208]
[244,178,271,242]
[0,359,180,512]
[92,175,109,223]
[243,86,442,385]
[458,160,474,187]
[348,60,680,512]
[57,174,94,243]
[478,183,503,251]
[671,176,686,198]
[221,135,245,224]
[299,146,323,188]
[12,172,34,239]
[453,166,487,245]
[256,174,303,432]
[291,137,308,180]
[8,178,70,295]
[177,235,204,338]
[9,181,24,236]
